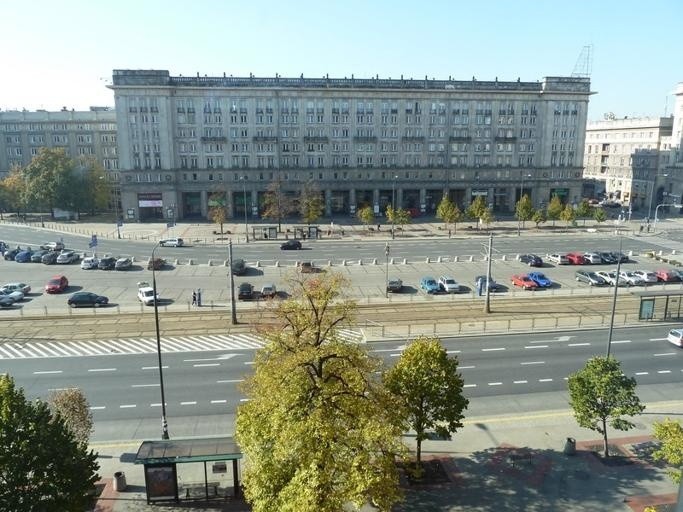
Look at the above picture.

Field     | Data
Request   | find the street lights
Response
[653,204,683,232]
[647,173,667,219]
[517,174,530,236]
[240,176,249,243]
[391,176,398,238]
[151,237,171,440]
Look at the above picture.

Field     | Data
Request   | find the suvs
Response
[237,283,254,300]
[68,292,108,308]
[136,281,160,306]
[261,283,276,297]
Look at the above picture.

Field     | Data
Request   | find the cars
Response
[510,248,683,291]
[231,259,246,275]
[0,238,184,272]
[403,208,421,217]
[0,284,30,306]
[587,198,620,208]
[386,274,500,293]
[45,275,68,293]
[280,240,301,250]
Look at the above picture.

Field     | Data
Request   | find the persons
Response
[196,288,201,306]
[191,290,197,306]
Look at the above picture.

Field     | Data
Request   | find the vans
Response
[666,329,683,347]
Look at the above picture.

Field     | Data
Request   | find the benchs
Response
[181,482,219,498]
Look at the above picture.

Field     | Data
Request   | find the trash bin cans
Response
[113,471,126,491]
[565,437,577,456]
